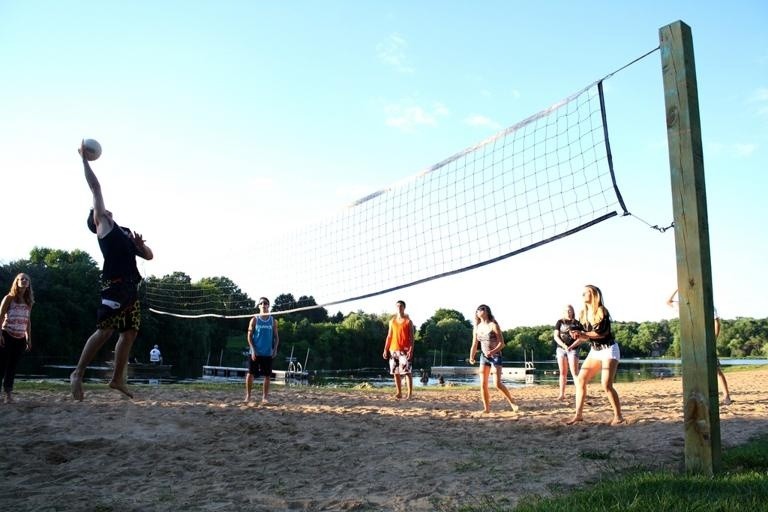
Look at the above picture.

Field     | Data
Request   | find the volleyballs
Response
[78,139,101,160]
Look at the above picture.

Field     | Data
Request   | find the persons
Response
[565,285,625,425]
[383,300,415,400]
[554,304,585,401]
[0,272,36,404]
[127,344,164,368]
[243,297,280,404]
[70,140,153,403]
[419,367,446,387]
[714,307,732,405]
[666,287,679,313]
[469,305,518,414]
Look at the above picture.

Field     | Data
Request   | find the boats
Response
[105,360,173,370]
